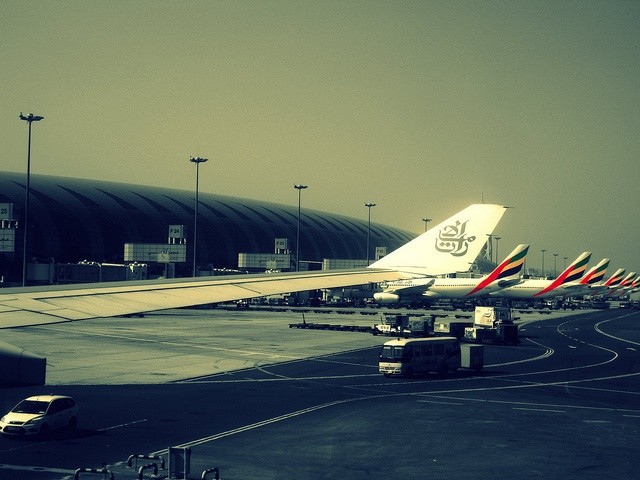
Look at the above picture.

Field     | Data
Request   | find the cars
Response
[1,393,79,440]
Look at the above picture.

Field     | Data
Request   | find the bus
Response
[378,337,461,377]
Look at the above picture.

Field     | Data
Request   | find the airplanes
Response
[487,251,591,312]
[578,258,610,311]
[613,272,637,308]
[372,244,529,309]
[0,204,507,386]
[631,275,640,305]
[599,268,626,309]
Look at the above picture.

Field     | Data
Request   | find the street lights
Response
[188,156,207,276]
[292,184,306,271]
[18,111,43,289]
[563,257,569,271]
[540,249,547,277]
[421,216,432,232]
[362,204,373,267]
[495,236,501,267]
[553,254,558,278]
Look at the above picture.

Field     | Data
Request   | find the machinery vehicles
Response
[369,313,413,338]
